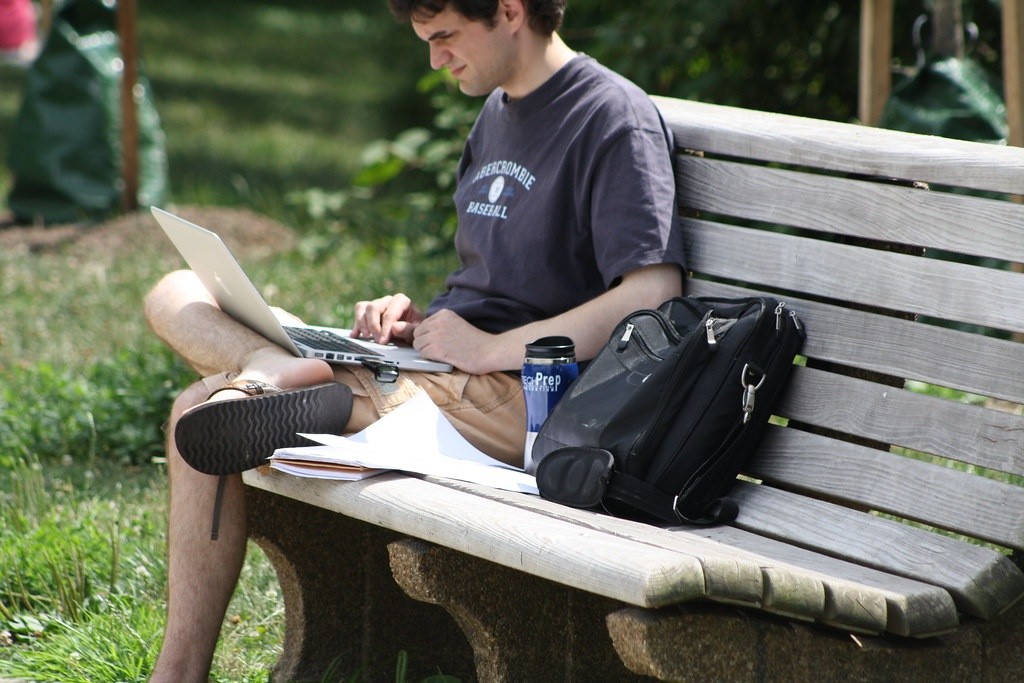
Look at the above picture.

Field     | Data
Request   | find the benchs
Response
[244,94,1024,683]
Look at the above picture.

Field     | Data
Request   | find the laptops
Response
[150,205,454,373]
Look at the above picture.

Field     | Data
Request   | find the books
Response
[268,446,392,483]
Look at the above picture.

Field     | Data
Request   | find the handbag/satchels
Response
[531,293,807,529]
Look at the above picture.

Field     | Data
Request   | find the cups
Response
[521,332,581,479]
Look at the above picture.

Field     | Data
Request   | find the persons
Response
[142,0,686,683]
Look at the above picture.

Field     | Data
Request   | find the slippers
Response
[174,380,353,476]
